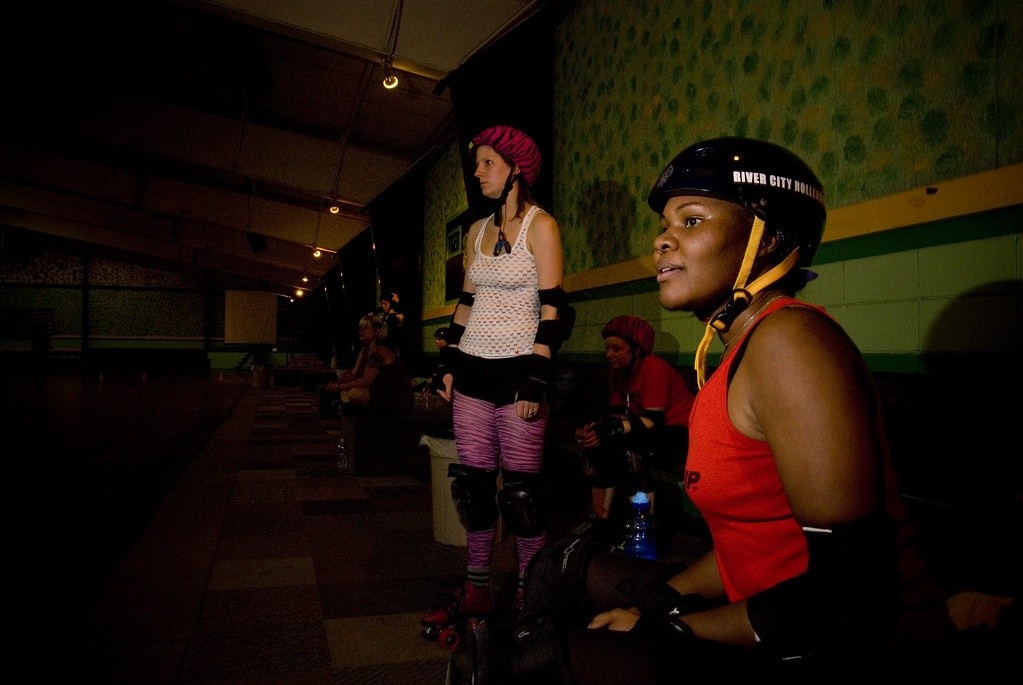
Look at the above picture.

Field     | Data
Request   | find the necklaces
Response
[724,295,787,347]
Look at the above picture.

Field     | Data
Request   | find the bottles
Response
[578,443,596,479]
[423,380,432,410]
[338,439,347,468]
[623,491,656,558]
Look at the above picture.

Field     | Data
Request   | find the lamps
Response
[380,62,398,89]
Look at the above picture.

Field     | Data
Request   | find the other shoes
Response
[567,515,611,543]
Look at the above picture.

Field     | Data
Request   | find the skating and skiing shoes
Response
[420,572,502,652]
[511,579,553,644]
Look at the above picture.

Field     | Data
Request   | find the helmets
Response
[434,327,449,338]
[380,290,400,306]
[648,137,826,268]
[600,314,656,354]
[467,125,542,188]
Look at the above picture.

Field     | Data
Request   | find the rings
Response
[578,440,580,443]
[528,412,534,414]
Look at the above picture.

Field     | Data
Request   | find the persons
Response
[422,125,564,638]
[368,291,404,358]
[575,315,693,517]
[414,327,453,401]
[329,316,415,404]
[449,137,962,685]
